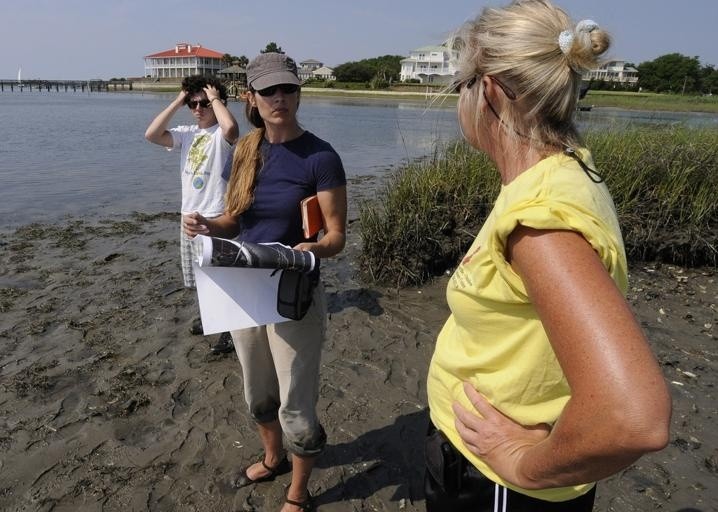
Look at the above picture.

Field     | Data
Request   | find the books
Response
[298,195,325,242]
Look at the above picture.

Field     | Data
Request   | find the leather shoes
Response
[235,451,288,488]
[279,482,314,512]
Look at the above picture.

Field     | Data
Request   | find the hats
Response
[246,51,301,91]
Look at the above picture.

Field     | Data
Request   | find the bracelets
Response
[206,96,225,110]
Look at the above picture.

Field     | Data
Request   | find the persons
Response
[177,47,347,511]
[424,0,674,511]
[144,73,243,354]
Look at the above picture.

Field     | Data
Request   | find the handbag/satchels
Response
[276,257,321,321]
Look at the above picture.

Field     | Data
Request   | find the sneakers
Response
[189,321,235,353]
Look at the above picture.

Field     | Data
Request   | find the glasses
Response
[187,97,225,109]
[453,69,518,101]
[253,90,299,96]
